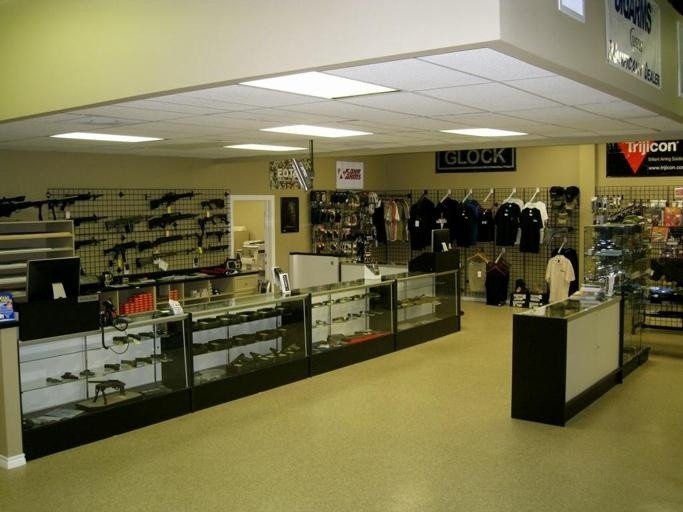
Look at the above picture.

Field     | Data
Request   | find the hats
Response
[549,187,563,199]
[565,185,578,203]
[563,200,577,210]
[551,198,564,208]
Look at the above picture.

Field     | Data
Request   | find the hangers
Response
[466,254,512,276]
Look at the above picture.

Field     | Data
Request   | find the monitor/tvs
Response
[432,229,452,251]
[26,256,80,305]
[291,157,309,191]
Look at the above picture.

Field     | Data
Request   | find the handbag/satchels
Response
[557,212,571,227]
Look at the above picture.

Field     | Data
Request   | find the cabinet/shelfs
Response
[0,220,75,303]
[584,223,645,296]
[100,265,261,325]
[19,268,461,463]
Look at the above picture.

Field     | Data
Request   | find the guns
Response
[0,191,228,268]
[45,370,94,383]
[86,380,125,404]
[113,329,168,344]
[104,354,168,371]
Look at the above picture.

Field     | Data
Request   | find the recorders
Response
[226,259,242,270]
